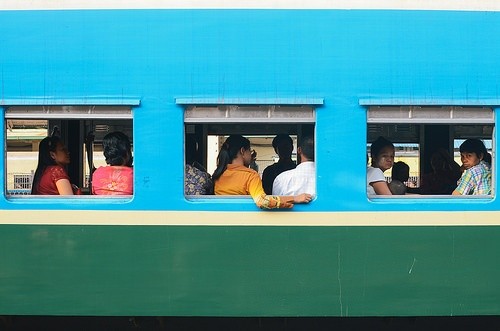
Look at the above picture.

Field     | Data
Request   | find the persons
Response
[91,131,133,195]
[272,132,315,196]
[185,138,213,195]
[245,150,259,173]
[31,136,81,195]
[389,161,409,194]
[423,138,490,196]
[213,134,312,209]
[262,134,296,195]
[367,136,395,195]
